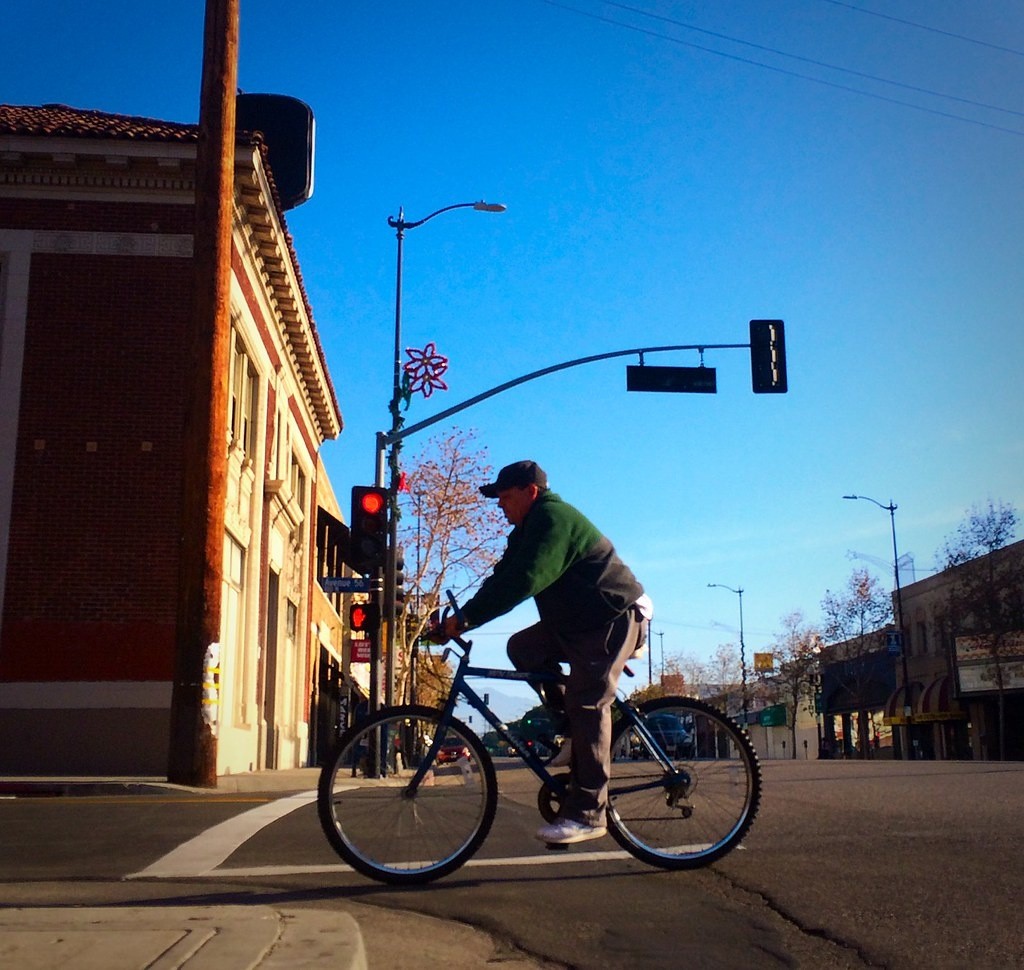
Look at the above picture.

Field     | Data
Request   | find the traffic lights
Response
[350,486,392,574]
[393,555,405,615]
[527,719,532,724]
[348,602,378,631]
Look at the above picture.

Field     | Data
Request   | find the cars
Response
[435,736,472,764]
[643,712,697,760]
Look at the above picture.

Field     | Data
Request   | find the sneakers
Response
[551,738,571,767]
[534,816,607,843]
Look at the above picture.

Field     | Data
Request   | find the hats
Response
[479,460,548,498]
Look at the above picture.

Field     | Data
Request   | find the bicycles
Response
[317,589,763,886]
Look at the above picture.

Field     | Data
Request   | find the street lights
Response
[842,493,916,760]
[378,199,507,776]
[706,582,748,734]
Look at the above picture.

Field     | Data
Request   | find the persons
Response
[429,460,648,845]
